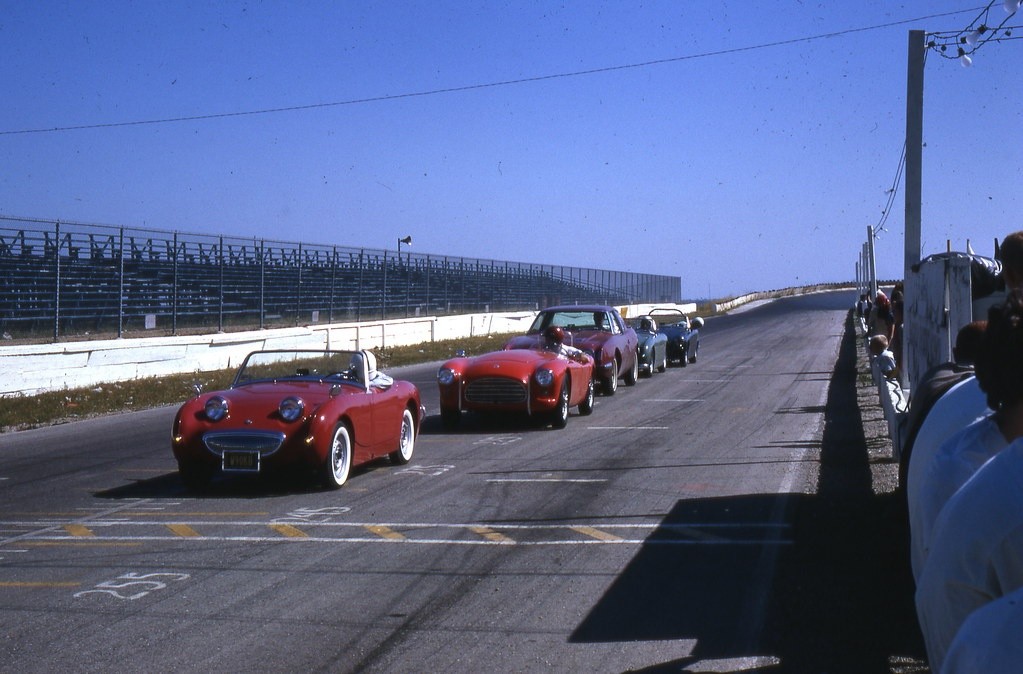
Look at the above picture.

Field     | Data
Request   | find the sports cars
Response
[438,341,597,430]
[648,309,704,365]
[624,319,669,379]
[170,347,426,492]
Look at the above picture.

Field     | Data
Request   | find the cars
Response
[501,304,641,395]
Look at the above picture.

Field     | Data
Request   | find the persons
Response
[902,230,1021,674]
[858,278,905,374]
[349,350,394,390]
[543,326,590,366]
[594,312,608,332]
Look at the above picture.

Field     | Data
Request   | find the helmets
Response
[349,348,377,377]
[639,315,656,332]
[691,315,703,328]
[543,326,565,345]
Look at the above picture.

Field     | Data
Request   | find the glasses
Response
[641,319,649,325]
